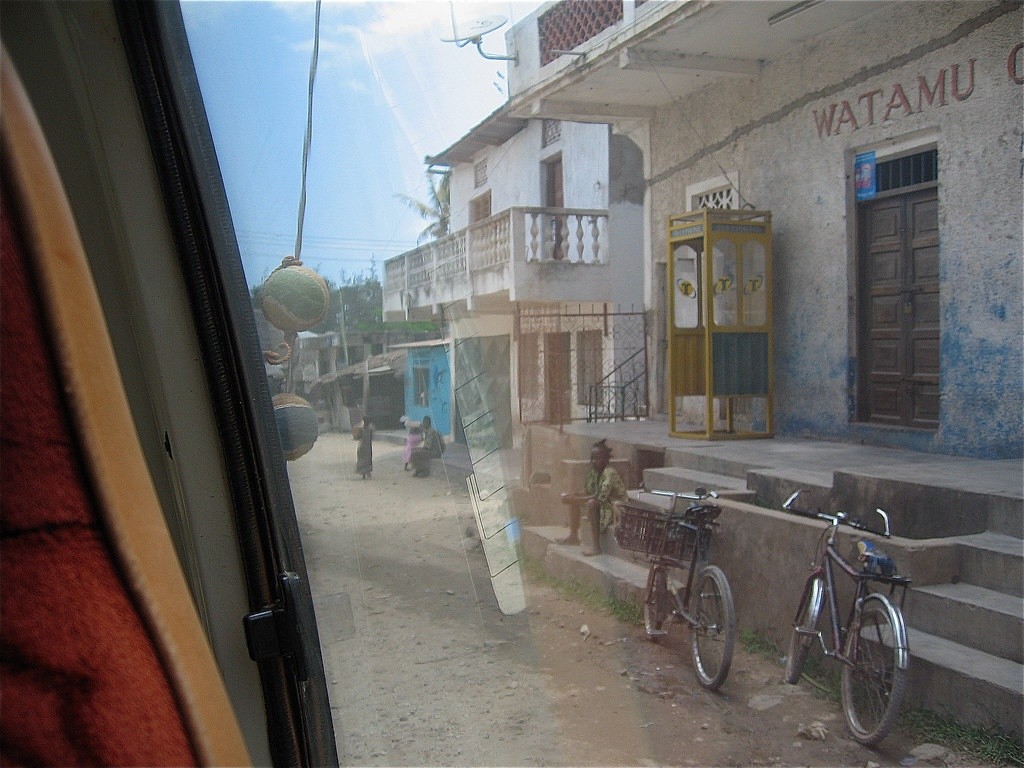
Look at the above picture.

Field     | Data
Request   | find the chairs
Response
[0,41,256,768]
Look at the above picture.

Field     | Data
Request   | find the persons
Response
[404,428,424,470]
[352,417,373,479]
[554,439,629,556]
[409,415,444,479]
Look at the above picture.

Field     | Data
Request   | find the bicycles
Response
[613,482,736,690]
[782,489,914,743]
